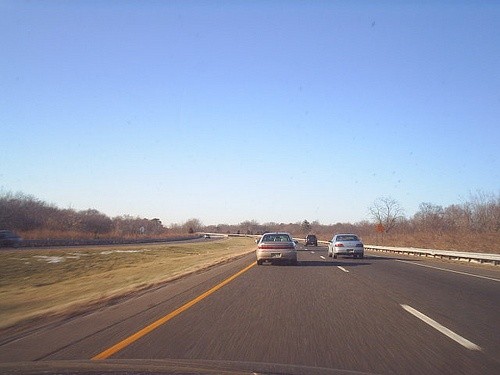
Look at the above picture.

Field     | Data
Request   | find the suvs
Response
[304,234,318,247]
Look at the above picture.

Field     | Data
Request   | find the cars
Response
[204,233,210,238]
[255,232,299,265]
[328,234,364,260]
